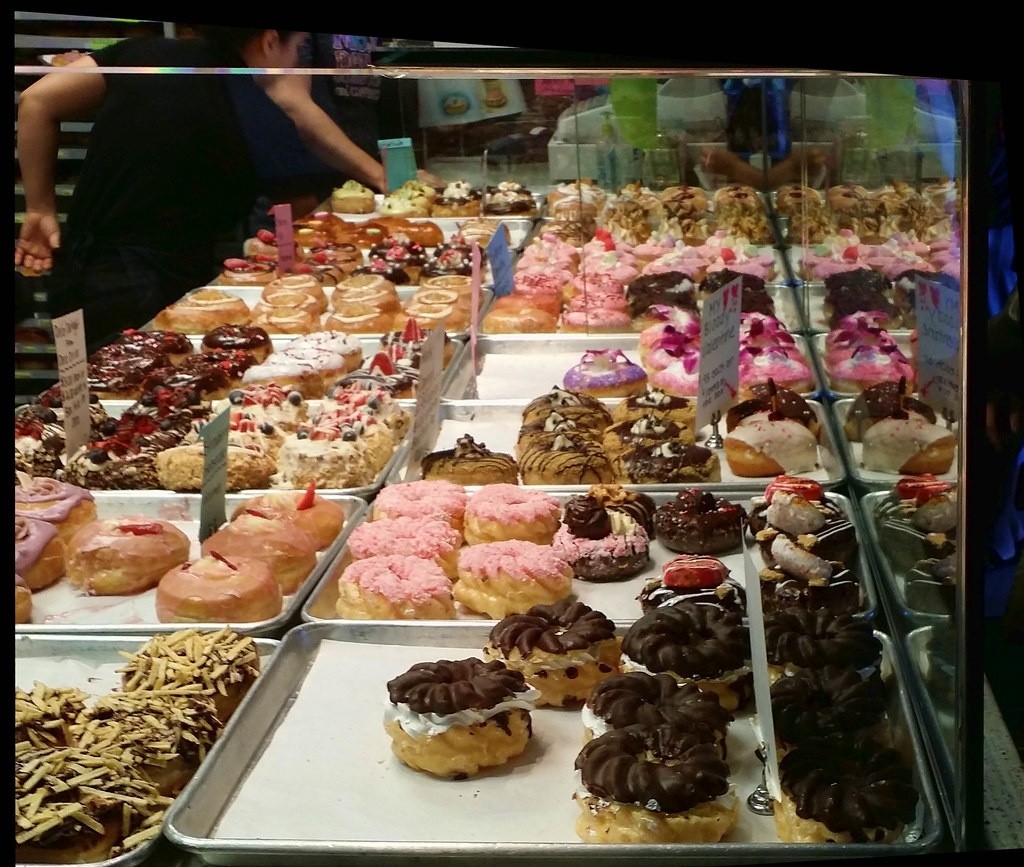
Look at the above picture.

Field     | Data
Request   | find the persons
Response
[14,22,432,363]
[699,75,962,410]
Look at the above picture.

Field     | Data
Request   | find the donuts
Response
[777,181,960,732]
[15,180,911,864]
